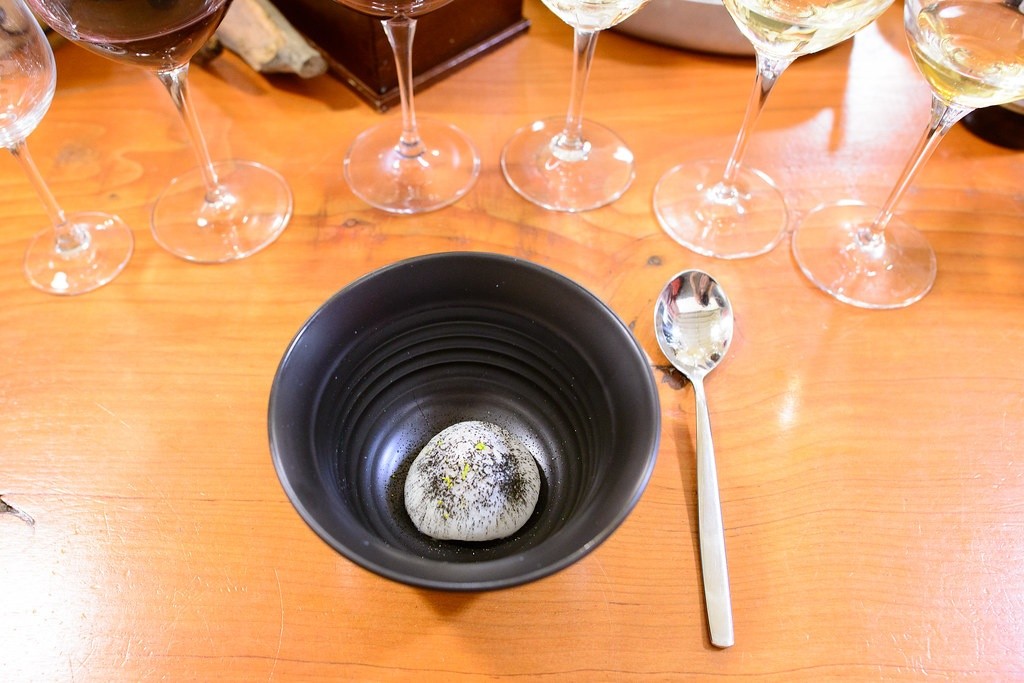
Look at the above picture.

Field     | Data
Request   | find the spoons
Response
[652,268,735,648]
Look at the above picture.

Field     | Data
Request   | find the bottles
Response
[957,0,1024,152]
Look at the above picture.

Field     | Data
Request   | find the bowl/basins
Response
[266,251,661,592]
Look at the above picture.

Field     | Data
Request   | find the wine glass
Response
[501,0,652,213]
[26,0,292,263]
[0,0,132,297]
[791,0,1024,313]
[652,0,897,260]
[332,0,481,215]
